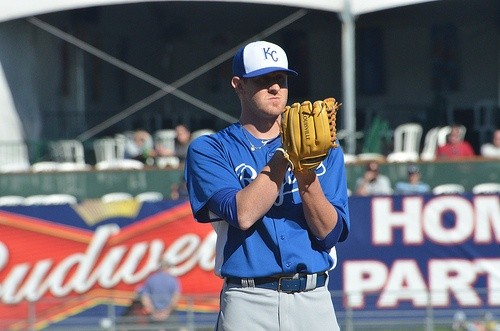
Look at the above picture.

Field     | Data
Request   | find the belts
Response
[226,274,326,291]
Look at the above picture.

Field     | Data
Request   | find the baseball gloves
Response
[280,98,343,175]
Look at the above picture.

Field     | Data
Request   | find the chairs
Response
[25,128,214,172]
[388,123,452,161]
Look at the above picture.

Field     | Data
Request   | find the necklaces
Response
[239,120,279,151]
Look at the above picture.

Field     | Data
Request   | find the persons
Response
[479,130,500,158]
[155,124,194,159]
[355,161,392,196]
[433,125,475,160]
[183,41,351,331]
[140,260,180,323]
[395,166,430,193]
[125,131,155,165]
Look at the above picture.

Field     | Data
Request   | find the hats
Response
[232,40,299,78]
